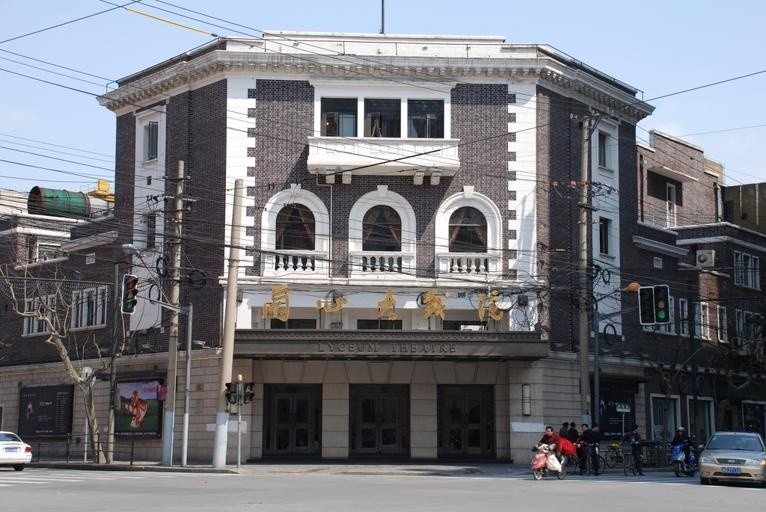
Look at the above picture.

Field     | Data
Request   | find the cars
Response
[698,431,766,485]
[0,431,33,470]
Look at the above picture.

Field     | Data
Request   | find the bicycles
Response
[565,443,643,476]
[530,444,569,480]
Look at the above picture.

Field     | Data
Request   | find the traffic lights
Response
[225,382,237,404]
[244,382,254,404]
[653,286,669,324]
[122,273,139,314]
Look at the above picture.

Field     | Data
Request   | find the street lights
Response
[123,243,180,467]
[79,372,94,462]
[579,281,642,431]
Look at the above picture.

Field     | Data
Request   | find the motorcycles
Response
[667,435,697,476]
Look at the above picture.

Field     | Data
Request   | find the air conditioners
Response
[695,247,716,269]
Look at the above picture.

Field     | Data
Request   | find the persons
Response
[537,422,601,476]
[669,427,696,468]
[618,424,645,476]
